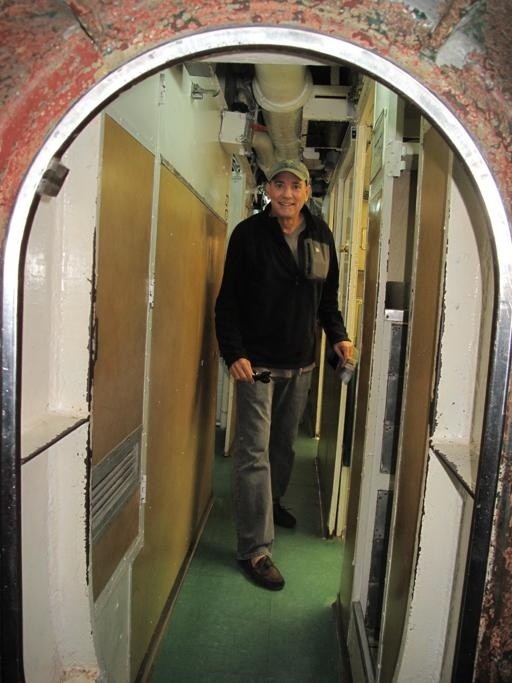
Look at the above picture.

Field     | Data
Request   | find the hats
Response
[267,159,309,183]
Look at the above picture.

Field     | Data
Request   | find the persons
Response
[214,156,351,590]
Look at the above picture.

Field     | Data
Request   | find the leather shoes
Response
[273,504,296,527]
[237,555,286,590]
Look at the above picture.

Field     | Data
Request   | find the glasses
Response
[251,368,272,384]
